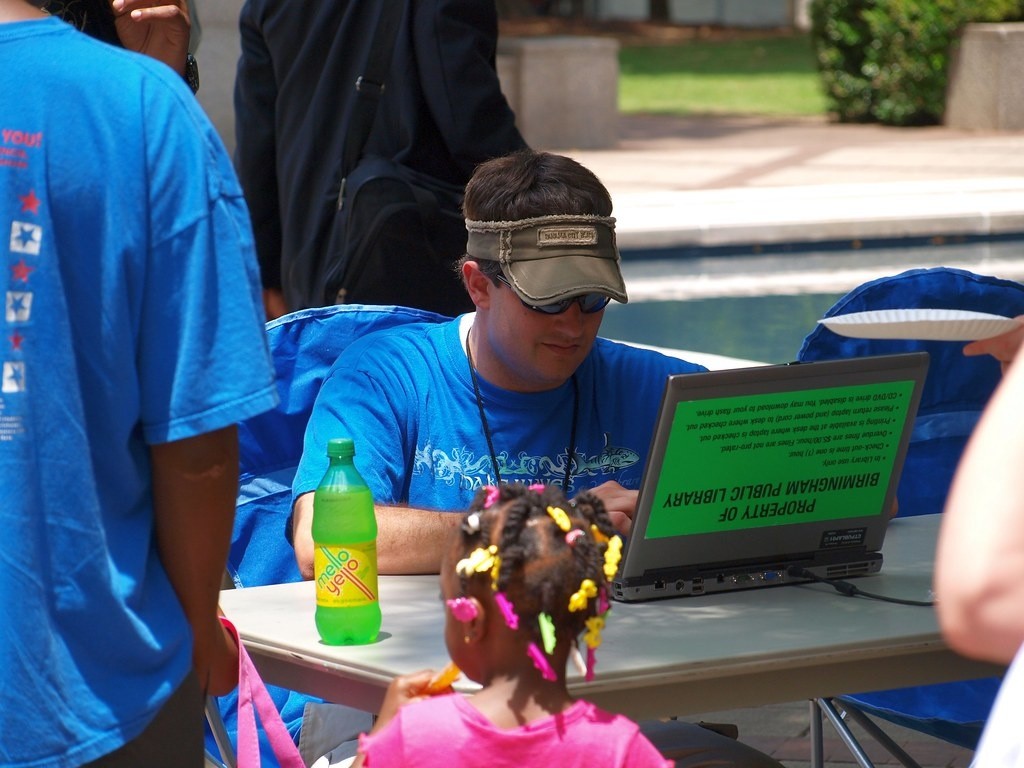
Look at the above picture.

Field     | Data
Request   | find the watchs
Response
[186,52,199,95]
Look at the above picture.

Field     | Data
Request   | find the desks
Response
[215,511,998,723]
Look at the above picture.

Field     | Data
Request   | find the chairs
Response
[795,268,1005,768]
[205,304,461,768]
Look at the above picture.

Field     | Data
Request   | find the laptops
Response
[606,353,933,605]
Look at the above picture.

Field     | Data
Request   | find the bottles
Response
[312,440,382,645]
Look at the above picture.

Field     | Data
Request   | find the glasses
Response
[495,275,611,315]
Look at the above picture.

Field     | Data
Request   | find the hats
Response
[465,218,629,306]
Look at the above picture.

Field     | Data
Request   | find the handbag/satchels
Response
[320,157,476,316]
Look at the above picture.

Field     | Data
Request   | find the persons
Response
[234,0,536,318]
[349,484,675,768]
[0,0,280,768]
[285,154,899,768]
[933,349,1024,768]
